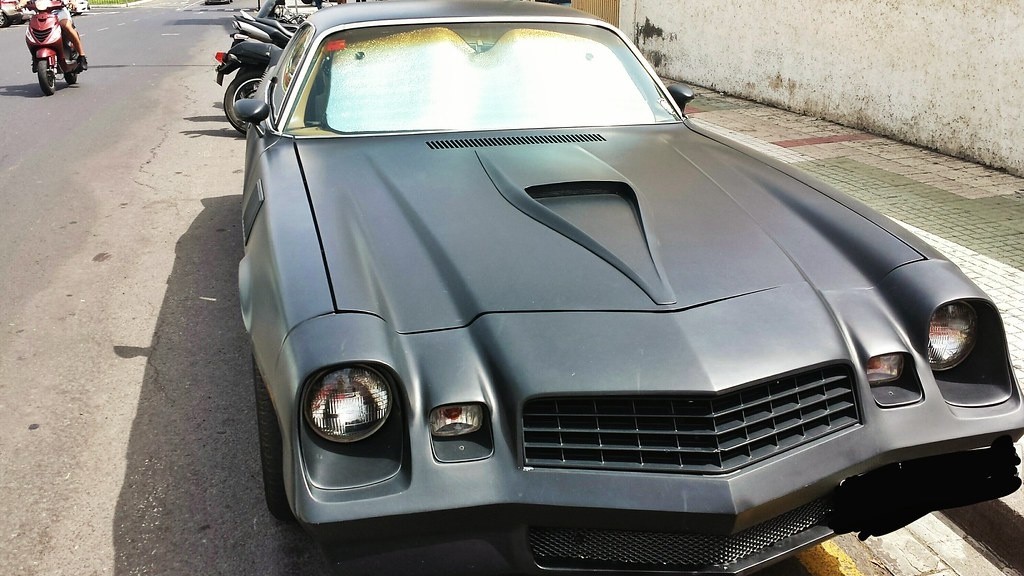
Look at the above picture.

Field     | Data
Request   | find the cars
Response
[204,0,234,4]
[233,0,1024,575]
[0,0,90,28]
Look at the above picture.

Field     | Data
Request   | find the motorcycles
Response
[14,0,88,96]
[214,0,314,135]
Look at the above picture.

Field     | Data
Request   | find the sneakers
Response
[76,54,88,70]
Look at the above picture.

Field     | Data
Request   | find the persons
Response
[14,0,88,72]
